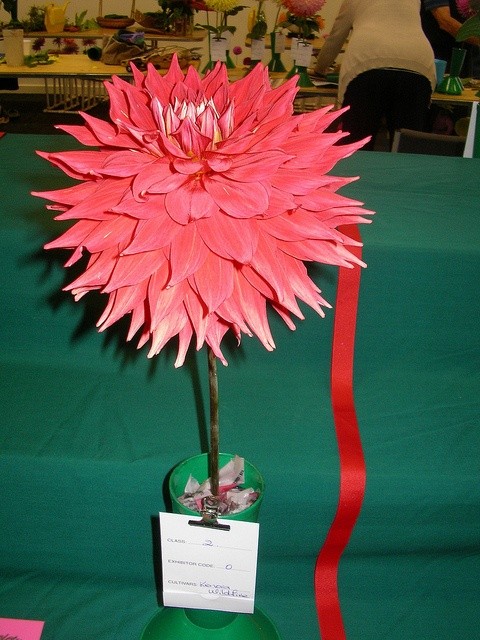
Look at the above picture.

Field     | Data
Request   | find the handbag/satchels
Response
[101,28,156,65]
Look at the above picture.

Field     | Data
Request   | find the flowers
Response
[31,51,371,523]
[158,0,325,85]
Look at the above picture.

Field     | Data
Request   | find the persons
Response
[420,0,479,74]
[314,0,439,155]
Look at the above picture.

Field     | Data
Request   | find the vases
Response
[168,452,264,523]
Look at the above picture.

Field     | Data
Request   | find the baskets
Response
[136,9,171,29]
[96,0,136,27]
